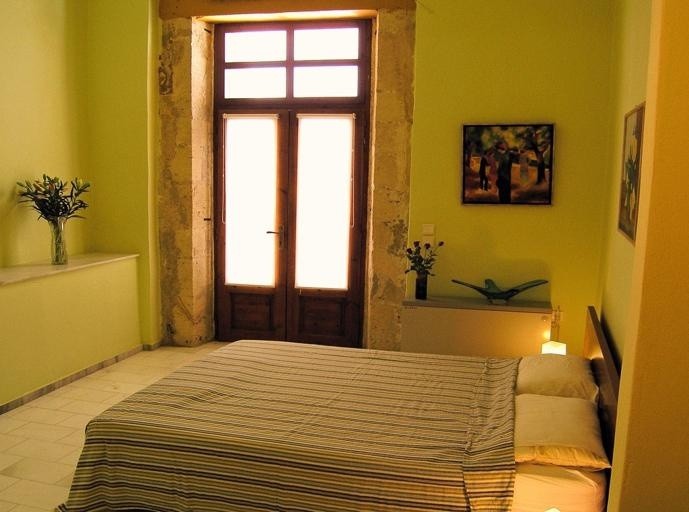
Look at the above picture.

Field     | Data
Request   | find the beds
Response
[53,302,620,509]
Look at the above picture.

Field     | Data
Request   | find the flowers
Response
[404,240,445,277]
[13,173,93,261]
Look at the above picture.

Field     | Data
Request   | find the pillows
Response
[475,352,613,476]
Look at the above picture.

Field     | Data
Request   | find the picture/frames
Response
[462,123,555,206]
[616,100,646,247]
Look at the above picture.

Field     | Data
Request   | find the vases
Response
[45,214,70,266]
[415,271,429,301]
